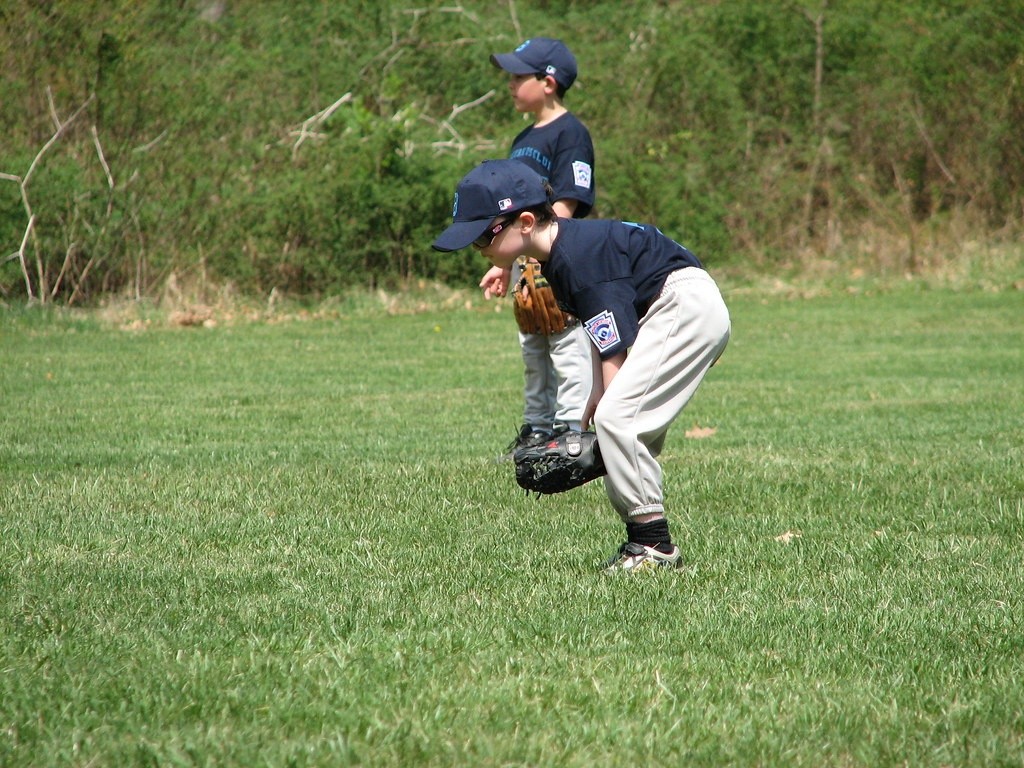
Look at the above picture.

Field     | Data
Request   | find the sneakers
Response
[502,424,556,455]
[600,542,684,574]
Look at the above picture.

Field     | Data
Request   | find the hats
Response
[432,159,551,252]
[489,37,577,89]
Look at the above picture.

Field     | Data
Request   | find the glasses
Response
[472,216,518,249]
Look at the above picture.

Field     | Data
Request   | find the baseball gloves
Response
[513,423,608,496]
[510,262,568,336]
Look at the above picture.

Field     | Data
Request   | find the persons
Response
[488,35,596,458]
[429,157,732,583]
